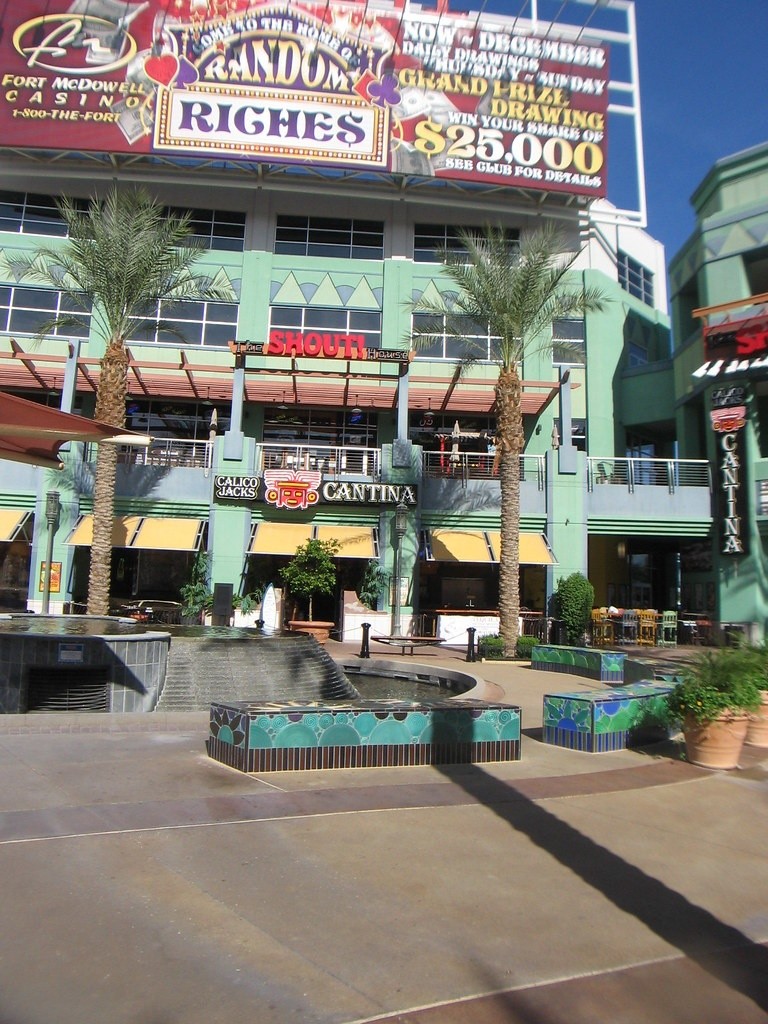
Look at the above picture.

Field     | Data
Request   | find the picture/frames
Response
[39,561,62,593]
[694,581,716,612]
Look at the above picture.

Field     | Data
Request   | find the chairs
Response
[65,600,185,625]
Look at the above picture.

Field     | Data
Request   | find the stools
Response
[519,607,712,648]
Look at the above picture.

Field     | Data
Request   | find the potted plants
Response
[662,623,768,771]
[278,536,345,646]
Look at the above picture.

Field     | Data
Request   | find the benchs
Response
[532,644,698,753]
[370,636,446,656]
[208,697,522,773]
